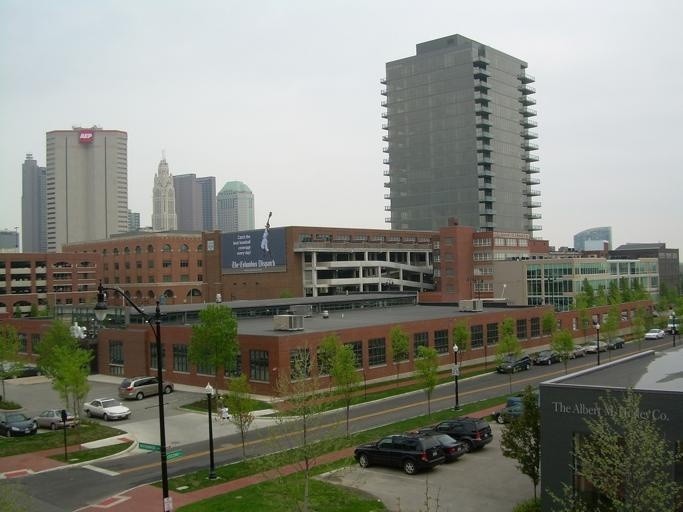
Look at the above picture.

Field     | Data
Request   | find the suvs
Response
[495,351,533,375]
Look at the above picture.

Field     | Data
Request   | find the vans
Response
[119,373,174,400]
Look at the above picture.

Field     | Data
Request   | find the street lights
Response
[451,343,463,412]
[205,384,217,480]
[671,311,676,347]
[596,322,601,366]
[92,281,169,511]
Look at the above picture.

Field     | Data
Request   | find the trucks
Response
[666,314,683,334]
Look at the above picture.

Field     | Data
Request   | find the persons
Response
[220,405,229,420]
[260,212,273,258]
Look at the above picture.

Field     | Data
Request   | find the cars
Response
[33,408,80,432]
[353,417,493,476]
[644,328,665,339]
[83,397,132,422]
[1,363,46,381]
[0,412,39,440]
[506,389,539,407]
[489,403,539,425]
[529,337,625,366]
